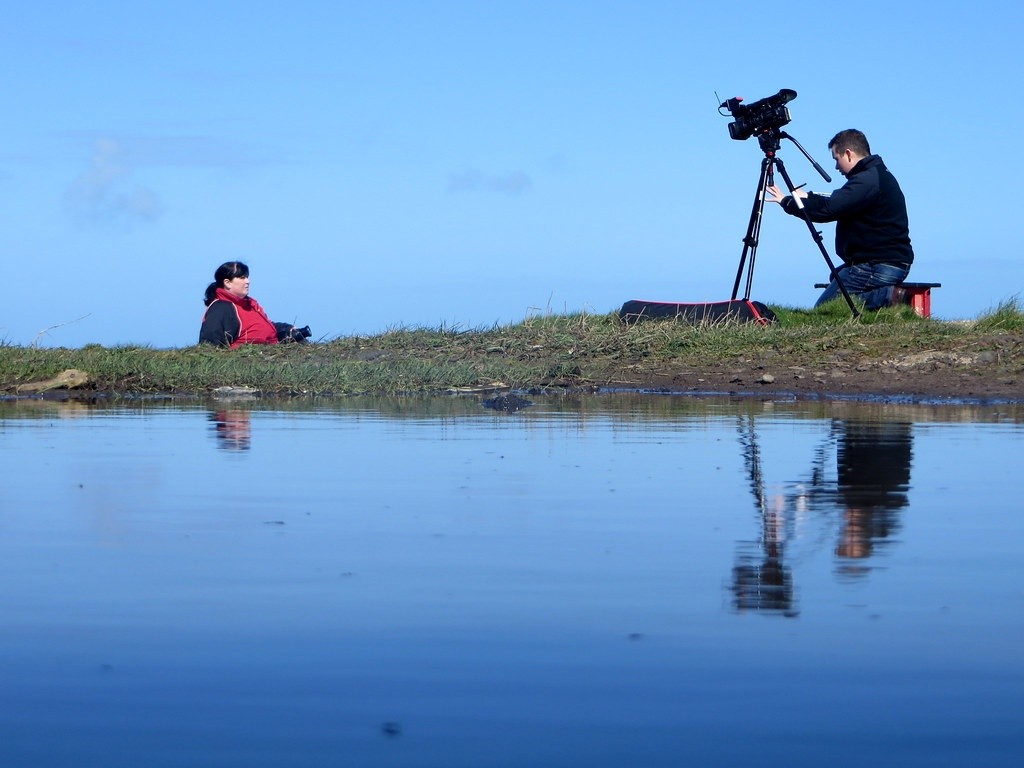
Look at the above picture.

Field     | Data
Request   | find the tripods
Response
[731,126,861,322]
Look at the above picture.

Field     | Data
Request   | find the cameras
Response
[286,326,312,344]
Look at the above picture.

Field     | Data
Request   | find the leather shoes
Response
[892,286,908,304]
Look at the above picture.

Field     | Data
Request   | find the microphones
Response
[722,97,743,106]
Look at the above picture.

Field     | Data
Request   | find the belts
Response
[884,260,910,270]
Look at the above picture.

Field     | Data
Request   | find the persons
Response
[199,261,295,348]
[766,128,915,312]
